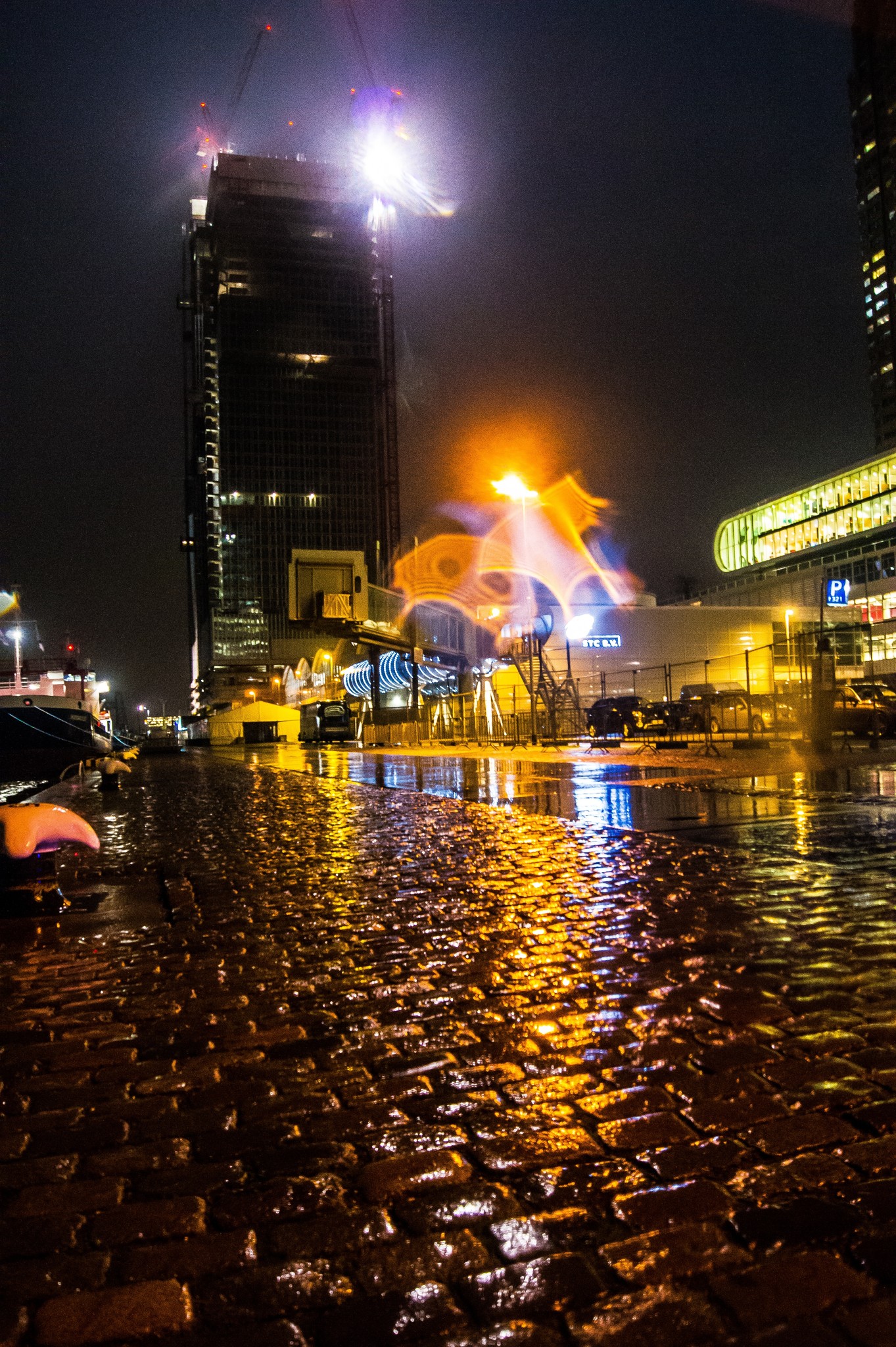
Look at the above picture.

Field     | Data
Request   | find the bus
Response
[298,700,354,745]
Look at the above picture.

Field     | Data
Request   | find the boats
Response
[0,581,116,778]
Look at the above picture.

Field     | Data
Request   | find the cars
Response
[648,703,705,734]
[832,685,896,739]
[705,694,798,734]
[586,696,652,738]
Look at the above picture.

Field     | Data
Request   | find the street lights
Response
[785,609,794,693]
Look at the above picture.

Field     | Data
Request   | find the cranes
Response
[196,21,274,153]
[337,1,393,114]
